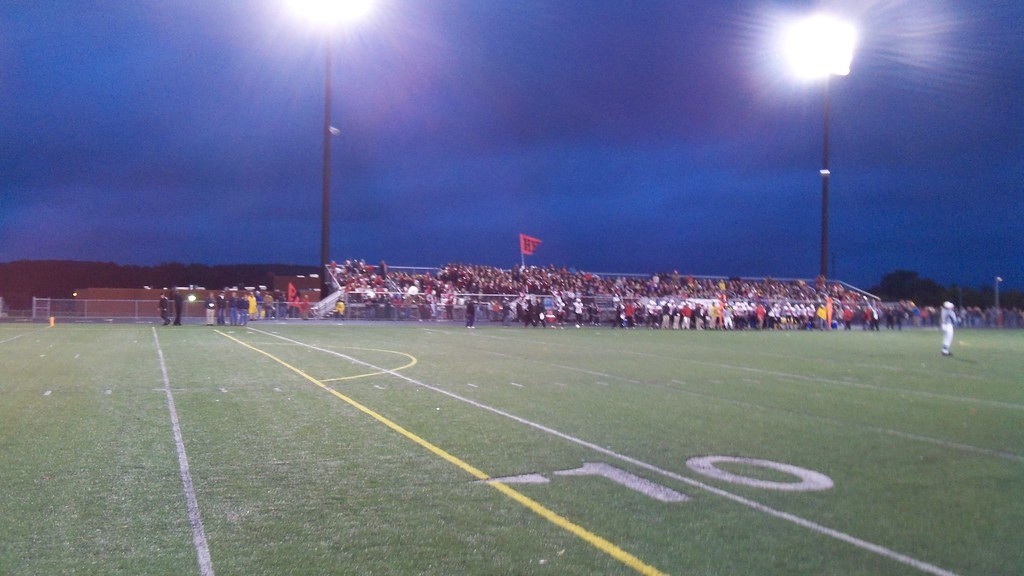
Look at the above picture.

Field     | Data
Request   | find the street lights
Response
[289,0,363,298]
[995,276,1003,308]
[801,18,855,279]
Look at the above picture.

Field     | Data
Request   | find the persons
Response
[216,292,226,324]
[940,301,957,356]
[955,306,1024,330]
[278,293,287,318]
[329,260,942,330]
[262,292,274,319]
[254,291,263,319]
[247,292,258,320]
[228,292,240,326]
[237,295,249,325]
[159,293,171,325]
[302,295,309,320]
[204,292,217,326]
[173,290,183,326]
